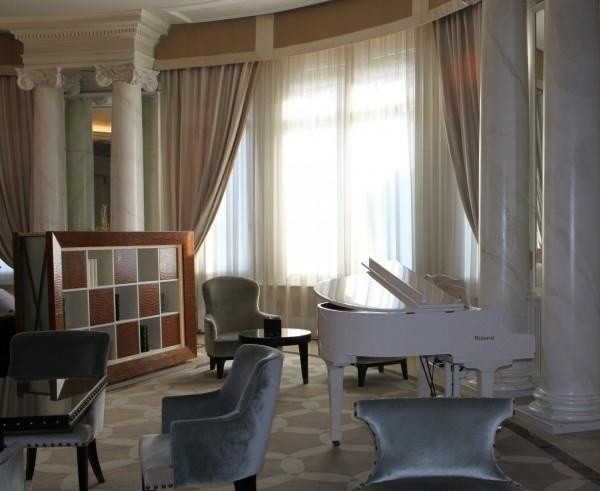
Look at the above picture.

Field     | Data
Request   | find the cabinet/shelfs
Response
[59,245,187,370]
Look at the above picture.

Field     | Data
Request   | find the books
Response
[115,293,120,321]
[141,325,151,353]
[87,258,98,289]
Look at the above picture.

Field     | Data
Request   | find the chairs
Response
[137,341,284,491]
[350,396,520,489]
[201,275,282,380]
[0,329,112,491]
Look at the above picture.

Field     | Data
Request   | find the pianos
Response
[313,257,535,446]
